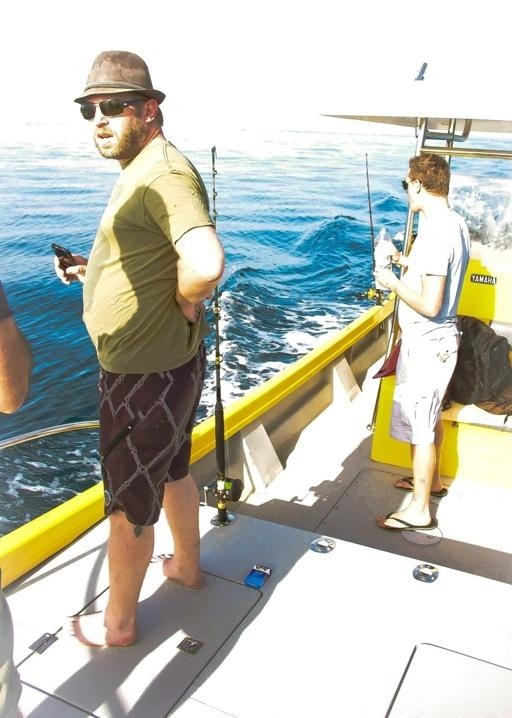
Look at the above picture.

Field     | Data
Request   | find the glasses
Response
[80,97,148,121]
[401,180,426,191]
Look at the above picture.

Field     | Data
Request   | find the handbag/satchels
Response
[451,314,511,406]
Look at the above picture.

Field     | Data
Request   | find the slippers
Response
[376,512,440,533]
[393,477,449,499]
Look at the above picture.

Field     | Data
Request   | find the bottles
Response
[372,224,393,292]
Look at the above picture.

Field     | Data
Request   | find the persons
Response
[1,286,33,716]
[372,151,471,532]
[52,49,227,650]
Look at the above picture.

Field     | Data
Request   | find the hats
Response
[74,51,167,105]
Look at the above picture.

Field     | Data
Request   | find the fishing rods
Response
[210,147,242,528]
[365,152,384,307]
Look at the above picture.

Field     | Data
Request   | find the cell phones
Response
[51,244,77,280]
